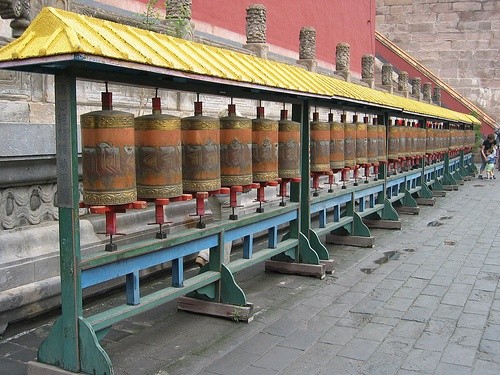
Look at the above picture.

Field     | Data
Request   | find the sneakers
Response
[488,176,493,179]
[483,176,490,180]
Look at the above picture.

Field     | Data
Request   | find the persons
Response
[479,134,498,180]
